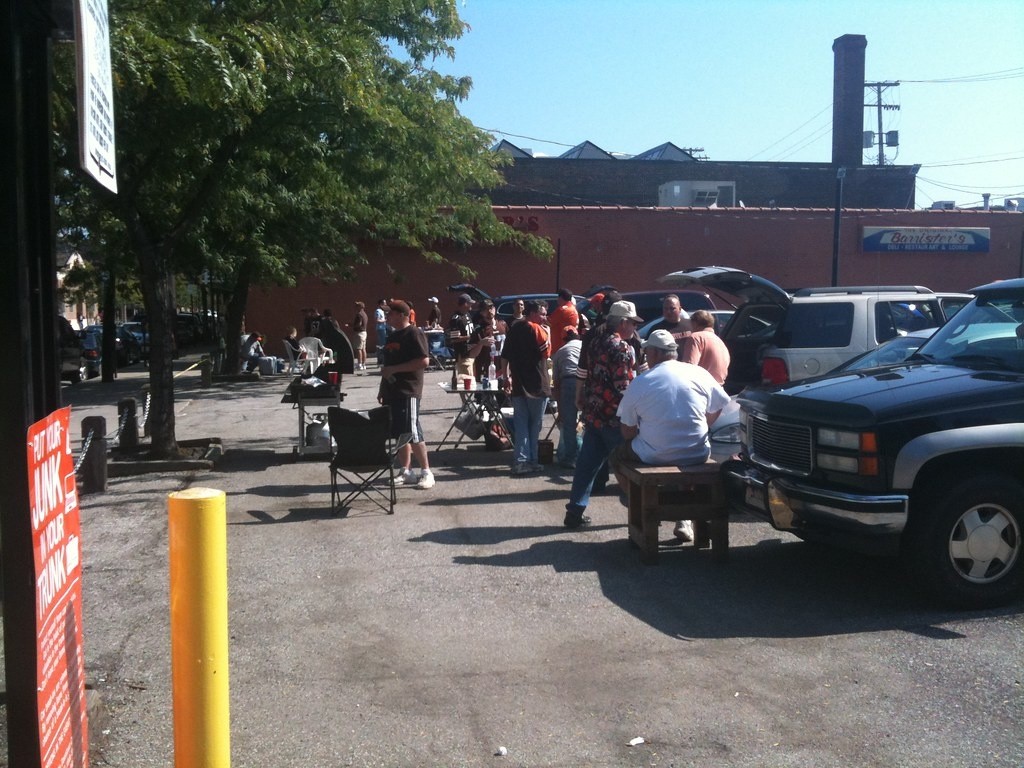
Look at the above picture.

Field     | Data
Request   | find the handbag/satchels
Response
[452,402,514,449]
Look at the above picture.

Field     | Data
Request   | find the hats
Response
[458,294,476,304]
[428,296,439,303]
[301,307,310,313]
[609,300,644,323]
[561,325,578,338]
[641,329,678,350]
[379,299,411,313]
[586,293,604,302]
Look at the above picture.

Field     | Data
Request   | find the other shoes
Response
[355,365,366,370]
[563,510,592,525]
[510,464,544,473]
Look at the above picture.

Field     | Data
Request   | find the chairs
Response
[326,405,399,520]
[282,337,337,381]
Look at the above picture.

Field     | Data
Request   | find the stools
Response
[616,456,730,564]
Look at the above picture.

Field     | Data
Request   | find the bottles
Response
[452,367,457,389]
[489,355,496,380]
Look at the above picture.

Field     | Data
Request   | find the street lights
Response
[832,168,847,285]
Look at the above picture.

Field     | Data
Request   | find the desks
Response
[280,372,347,456]
[419,328,446,371]
[433,383,524,455]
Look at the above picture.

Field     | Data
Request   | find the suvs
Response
[717,277,1024,609]
[658,265,975,387]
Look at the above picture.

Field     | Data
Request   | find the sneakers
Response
[388,469,417,486]
[673,519,694,541]
[417,469,435,489]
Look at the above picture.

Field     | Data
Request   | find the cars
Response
[702,322,1023,466]
[446,282,588,321]
[58,310,220,379]
[635,309,772,340]
[576,290,718,338]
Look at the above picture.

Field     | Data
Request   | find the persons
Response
[285,326,310,372]
[552,325,582,468]
[575,291,650,494]
[682,310,730,387]
[610,329,731,541]
[444,294,475,361]
[407,302,415,325]
[500,300,551,474]
[576,293,605,315]
[505,298,525,335]
[377,300,435,489]
[466,299,504,404]
[354,301,368,370]
[374,297,397,365]
[546,288,579,358]
[305,308,338,356]
[427,296,441,326]
[564,301,644,525]
[647,294,694,362]
[245,337,265,372]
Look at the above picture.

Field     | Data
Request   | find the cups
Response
[328,372,337,385]
[464,379,471,389]
